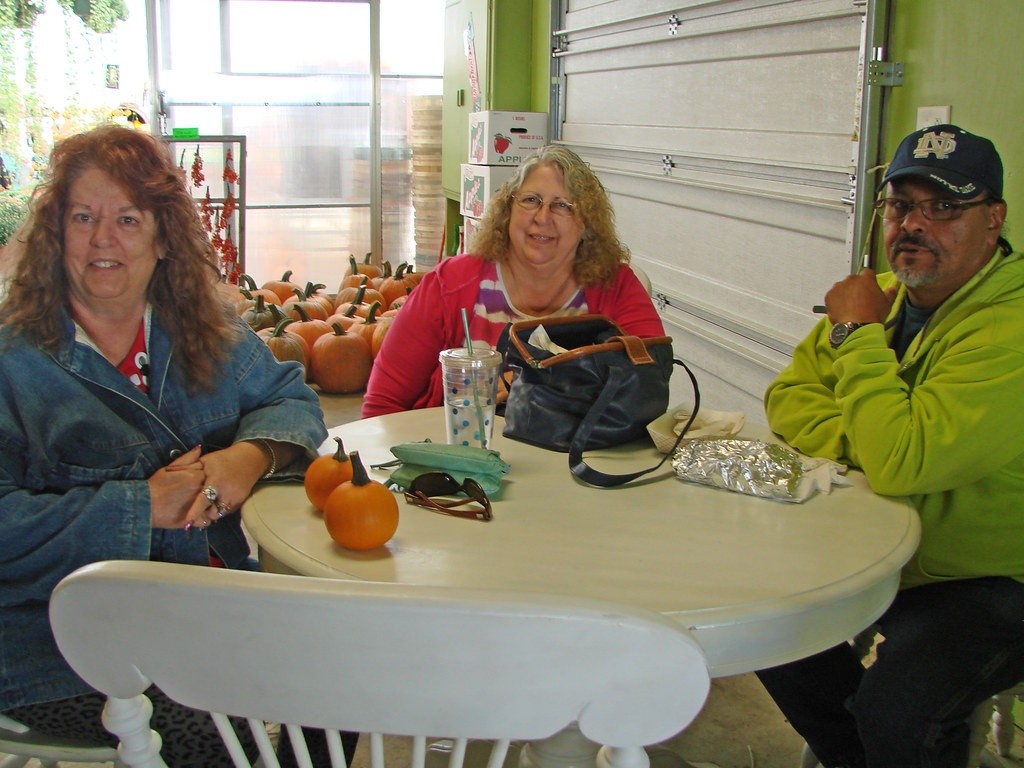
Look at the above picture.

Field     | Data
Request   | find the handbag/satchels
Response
[371,440,508,496]
[506,313,697,486]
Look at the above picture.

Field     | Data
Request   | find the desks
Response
[240,405,922,768]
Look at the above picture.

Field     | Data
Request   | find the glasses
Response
[404,472,493,521]
[510,194,577,216]
[872,197,990,220]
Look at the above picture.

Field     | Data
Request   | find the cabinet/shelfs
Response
[440,0,533,258]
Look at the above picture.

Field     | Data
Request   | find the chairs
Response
[0,714,119,768]
[49,560,712,768]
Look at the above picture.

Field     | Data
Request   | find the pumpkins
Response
[324,450,400,552]
[213,252,427,393]
[304,437,355,512]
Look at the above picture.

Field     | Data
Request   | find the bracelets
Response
[258,438,277,478]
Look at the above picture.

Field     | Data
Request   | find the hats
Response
[876,124,1003,200]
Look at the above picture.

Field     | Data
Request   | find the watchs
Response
[829,320,871,348]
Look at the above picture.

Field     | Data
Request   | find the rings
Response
[200,521,207,531]
[202,486,217,503]
[214,501,232,518]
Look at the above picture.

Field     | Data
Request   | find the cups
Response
[439,347,503,449]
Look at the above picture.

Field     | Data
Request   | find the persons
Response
[754,124,1024,768]
[361,144,667,420]
[0,125,360,768]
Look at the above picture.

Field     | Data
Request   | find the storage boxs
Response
[468,109,548,166]
[463,217,480,254]
[460,163,518,219]
